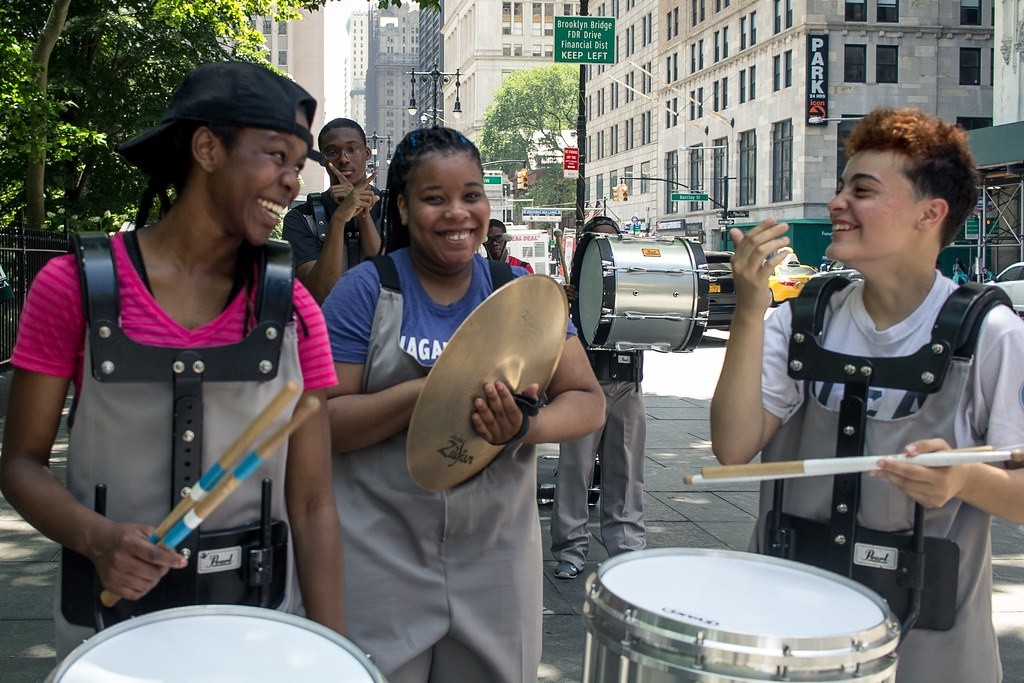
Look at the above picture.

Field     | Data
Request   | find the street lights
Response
[404,68,462,128]
[371,136,391,187]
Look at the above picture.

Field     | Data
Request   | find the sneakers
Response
[554,560,578,578]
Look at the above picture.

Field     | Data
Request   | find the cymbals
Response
[405,273,568,490]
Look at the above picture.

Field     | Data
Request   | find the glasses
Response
[485,235,504,244]
[324,146,366,160]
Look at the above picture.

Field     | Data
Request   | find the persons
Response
[282,119,391,308]
[0,60,347,644]
[318,126,607,683]
[483,219,534,273]
[550,215,646,578]
[710,106,1024,683]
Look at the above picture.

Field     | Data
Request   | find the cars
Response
[702,250,736,332]
[766,265,818,307]
[810,260,863,283]
[985,262,1024,311]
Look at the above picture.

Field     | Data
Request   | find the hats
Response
[115,61,316,175]
[584,217,620,233]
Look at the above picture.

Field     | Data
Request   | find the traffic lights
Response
[517,170,528,189]
[612,184,628,202]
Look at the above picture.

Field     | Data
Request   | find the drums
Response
[51,603,388,683]
[537,454,602,507]
[572,231,710,353]
[585,546,902,683]
